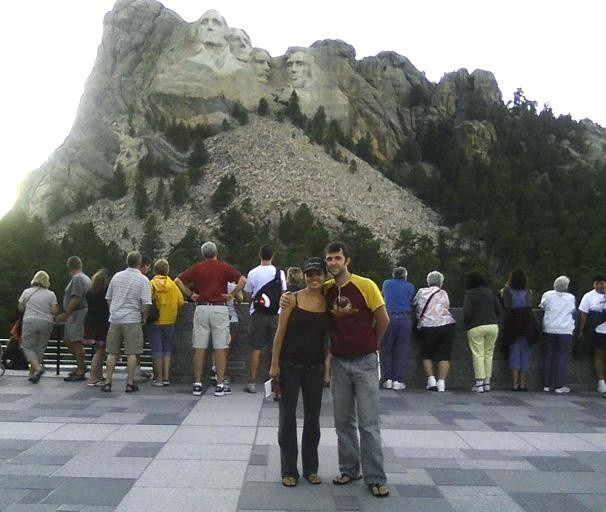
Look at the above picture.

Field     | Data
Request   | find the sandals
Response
[125,383,138,392]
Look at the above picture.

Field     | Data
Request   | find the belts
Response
[197,301,226,305]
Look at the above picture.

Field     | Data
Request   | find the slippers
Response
[369,483,389,497]
[282,474,321,486]
[87,378,111,392]
[333,474,362,484]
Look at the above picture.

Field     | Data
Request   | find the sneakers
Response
[382,379,406,390]
[192,383,203,396]
[65,374,84,381]
[29,368,44,383]
[426,378,445,392]
[471,383,490,393]
[210,366,231,396]
[555,387,569,393]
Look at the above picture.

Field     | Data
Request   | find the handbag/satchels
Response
[416,321,423,332]
[148,304,159,322]
[253,265,282,313]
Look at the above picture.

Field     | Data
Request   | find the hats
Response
[303,257,323,274]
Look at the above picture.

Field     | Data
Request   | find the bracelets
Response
[579,329,585,332]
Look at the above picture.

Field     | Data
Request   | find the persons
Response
[537,275,578,394]
[243,244,333,394]
[267,257,333,487]
[500,267,538,392]
[250,47,273,85]
[58,256,111,387]
[173,241,246,395]
[284,48,312,88]
[227,27,252,65]
[411,270,458,392]
[197,9,226,47]
[381,266,419,390]
[13,269,59,382]
[102,252,183,393]
[279,241,391,498]
[577,271,606,394]
[463,271,503,393]
